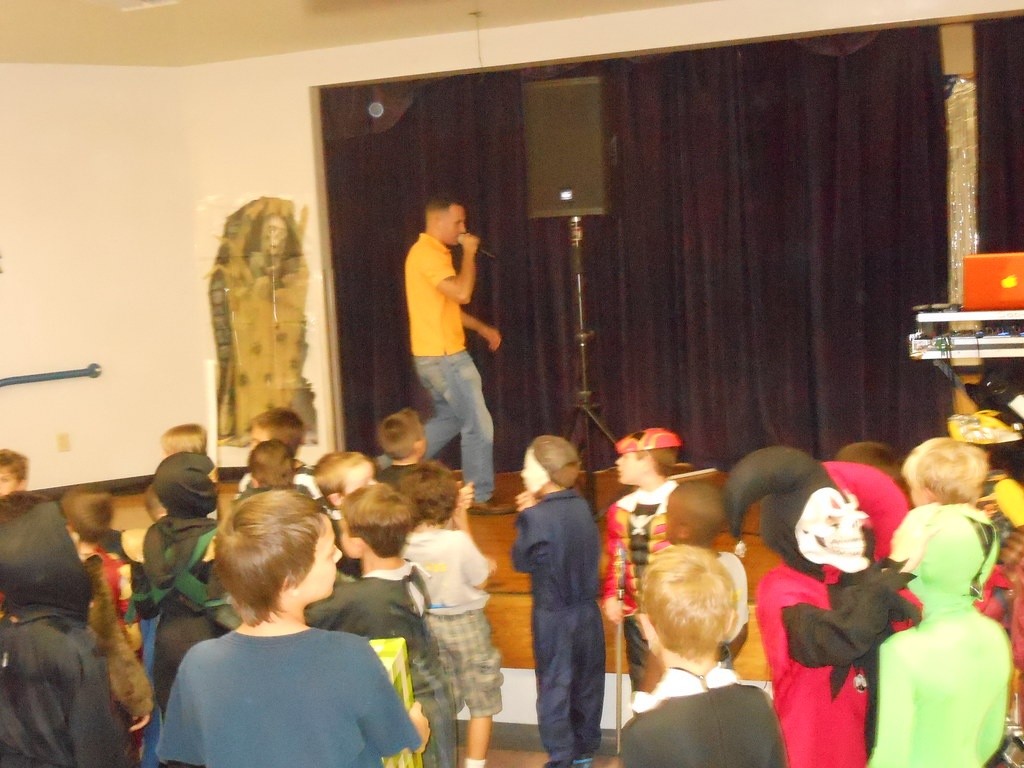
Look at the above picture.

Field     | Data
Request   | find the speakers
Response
[520,75,609,220]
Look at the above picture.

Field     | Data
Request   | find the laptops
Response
[962,252,1023,311]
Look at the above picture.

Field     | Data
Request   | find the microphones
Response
[457,233,497,259]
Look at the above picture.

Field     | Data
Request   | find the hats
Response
[532,433,583,487]
[614,428,683,454]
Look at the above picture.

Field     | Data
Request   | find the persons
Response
[514,435,606,768]
[0,407,506,768]
[602,427,1024,768]
[372,190,515,515]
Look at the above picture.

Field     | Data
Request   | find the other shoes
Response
[468,500,519,515]
[543,758,593,768]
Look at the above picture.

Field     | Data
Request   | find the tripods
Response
[565,216,634,523]
